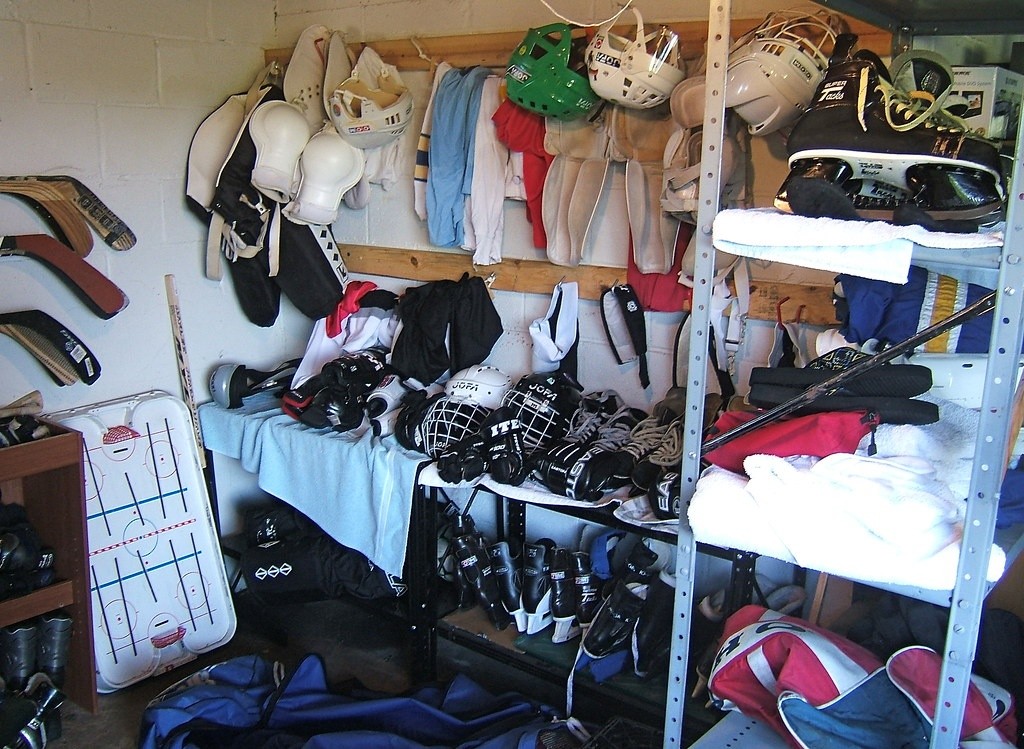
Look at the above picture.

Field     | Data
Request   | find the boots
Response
[0,612,73,692]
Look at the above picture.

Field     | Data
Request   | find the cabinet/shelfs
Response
[665,0,1024,749]
[0,415,99,714]
[431,469,760,740]
[198,390,436,684]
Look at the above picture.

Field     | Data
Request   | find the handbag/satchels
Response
[708,605,1017,749]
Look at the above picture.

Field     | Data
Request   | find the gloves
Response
[437,407,527,487]
[321,346,392,428]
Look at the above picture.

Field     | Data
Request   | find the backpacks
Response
[240,504,408,605]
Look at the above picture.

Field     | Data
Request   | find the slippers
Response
[748,348,939,426]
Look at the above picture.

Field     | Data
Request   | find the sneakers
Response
[774,32,1007,234]
[528,387,723,521]
[452,513,806,707]
[0,414,51,449]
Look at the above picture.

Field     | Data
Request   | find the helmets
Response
[445,365,586,419]
[0,487,55,601]
[584,15,687,108]
[504,22,601,122]
[725,29,822,136]
[330,88,415,149]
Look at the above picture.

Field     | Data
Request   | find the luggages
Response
[47,389,237,693]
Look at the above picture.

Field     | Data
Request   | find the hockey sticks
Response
[0,174,138,388]
[164,275,220,532]
